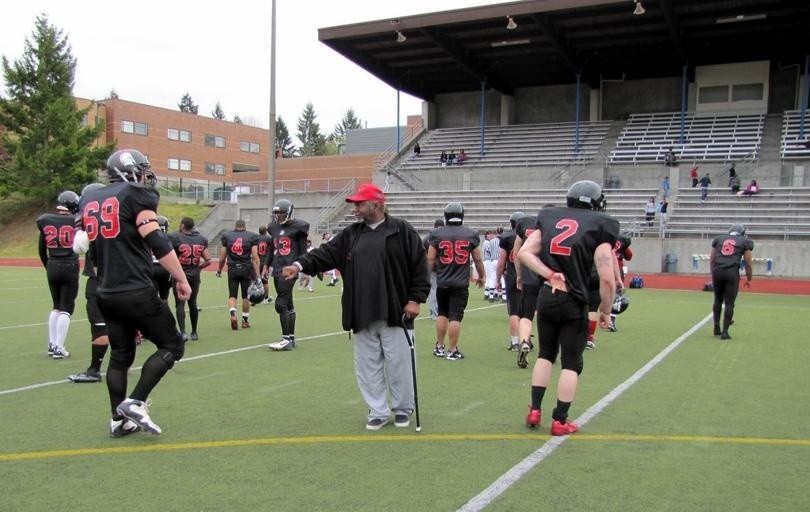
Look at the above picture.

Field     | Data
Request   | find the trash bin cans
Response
[666,254,677,272]
[609,177,619,193]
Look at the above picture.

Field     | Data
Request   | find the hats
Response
[345,182,386,204]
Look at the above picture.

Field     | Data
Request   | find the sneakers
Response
[268,339,293,353]
[365,419,389,431]
[116,398,164,436]
[110,417,143,439]
[240,320,251,328]
[433,342,446,358]
[46,343,71,360]
[525,408,542,428]
[190,330,199,342]
[482,291,508,306]
[445,347,465,361]
[229,313,238,331]
[550,418,580,436]
[393,414,410,428]
[66,368,104,384]
[516,339,530,370]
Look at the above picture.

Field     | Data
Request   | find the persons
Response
[585,220,632,350]
[216,200,340,350]
[687,164,700,188]
[281,182,431,431]
[440,147,466,167]
[519,180,616,434]
[68,184,111,384]
[664,148,678,167]
[728,162,761,197]
[422,203,556,369]
[708,224,753,339]
[135,216,212,345]
[80,149,193,439]
[411,142,422,160]
[646,177,671,229]
[36,191,82,359]
[696,173,712,202]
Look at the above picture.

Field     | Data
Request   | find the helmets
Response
[727,222,747,236]
[247,282,266,305]
[271,198,296,225]
[510,209,526,230]
[565,181,605,210]
[156,214,171,230]
[442,201,466,222]
[105,148,152,185]
[612,294,631,315]
[54,190,82,215]
